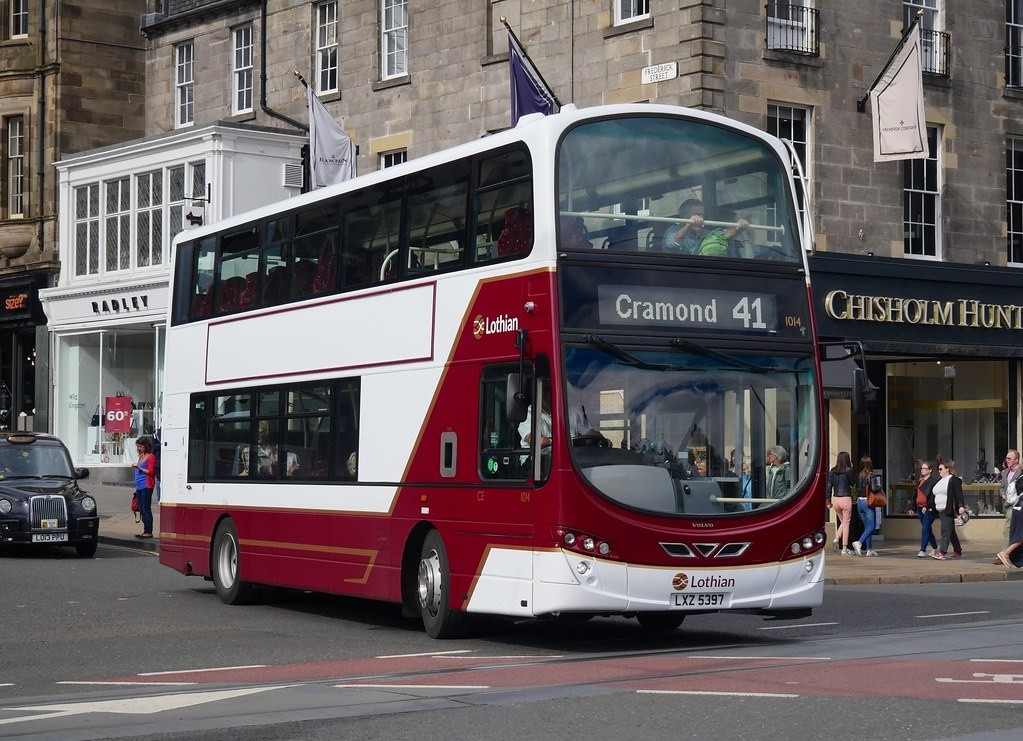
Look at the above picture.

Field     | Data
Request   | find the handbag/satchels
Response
[866,474,887,506]
[132,492,140,512]
[954,511,970,526]
[1004,474,1023,507]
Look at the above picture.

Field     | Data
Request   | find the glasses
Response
[936,468,946,471]
[921,467,929,471]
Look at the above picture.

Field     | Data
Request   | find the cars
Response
[0,432,99,559]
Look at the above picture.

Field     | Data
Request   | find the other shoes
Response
[135,533,153,539]
[832,540,839,552]
[932,552,945,560]
[841,549,856,556]
[993,557,1004,565]
[948,552,963,559]
[866,550,878,557]
[917,551,926,558]
[852,541,862,556]
[996,551,1012,569]
[928,549,939,557]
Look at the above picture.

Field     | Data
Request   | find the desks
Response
[890,480,1002,514]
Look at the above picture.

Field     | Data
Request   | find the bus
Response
[161,104,878,639]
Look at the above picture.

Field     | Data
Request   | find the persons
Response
[908,462,938,559]
[851,458,882,557]
[765,447,790,499]
[131,436,156,539]
[286,451,300,478]
[347,453,357,478]
[661,199,748,257]
[687,427,750,510]
[242,418,280,474]
[991,449,1023,569]
[516,379,613,482]
[931,457,965,559]
[827,452,857,556]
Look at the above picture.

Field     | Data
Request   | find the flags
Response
[305,86,358,190]
[508,34,555,128]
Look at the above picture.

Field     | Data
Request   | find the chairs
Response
[233,443,249,474]
[497,208,672,258]
[193,246,419,317]
